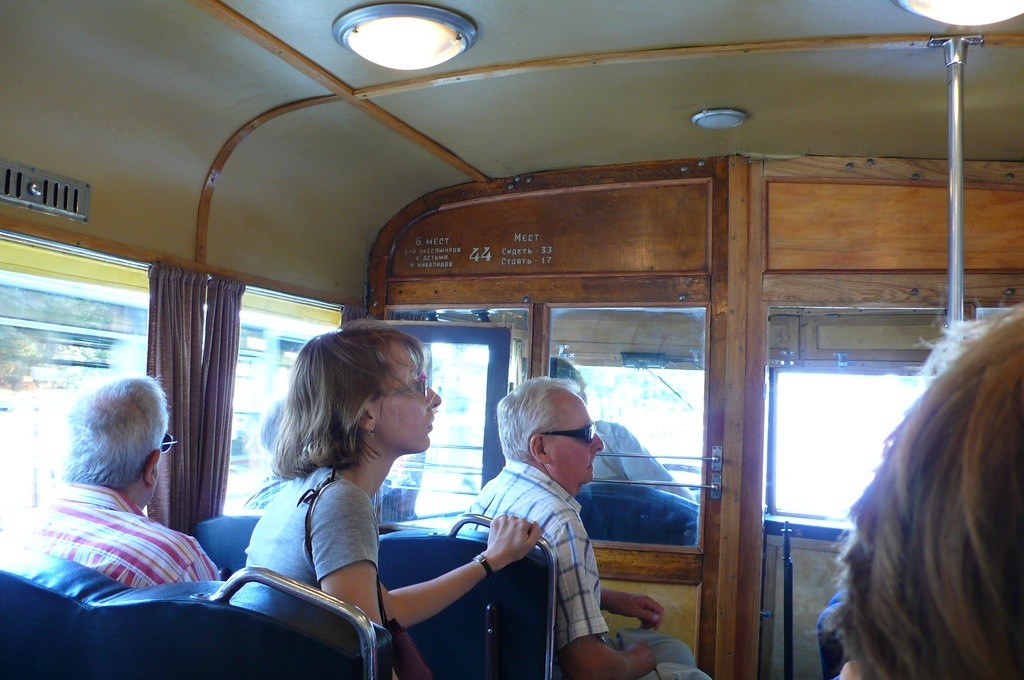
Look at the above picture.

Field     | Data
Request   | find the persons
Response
[24,374,219,589]
[464,376,712,680]
[548,357,697,501]
[817,303,1024,680]
[243,317,540,680]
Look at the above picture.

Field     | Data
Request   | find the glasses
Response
[371,376,428,399]
[157,433,177,454]
[540,422,597,443]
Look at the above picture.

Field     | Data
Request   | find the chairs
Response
[575,482,698,546]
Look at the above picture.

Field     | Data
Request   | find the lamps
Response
[331,2,477,71]
[890,0,1024,26]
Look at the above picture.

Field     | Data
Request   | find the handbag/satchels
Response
[297,463,432,680]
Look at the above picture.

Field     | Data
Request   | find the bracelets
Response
[475,555,492,578]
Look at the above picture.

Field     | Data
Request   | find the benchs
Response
[0,549,395,680]
[194,514,557,680]
